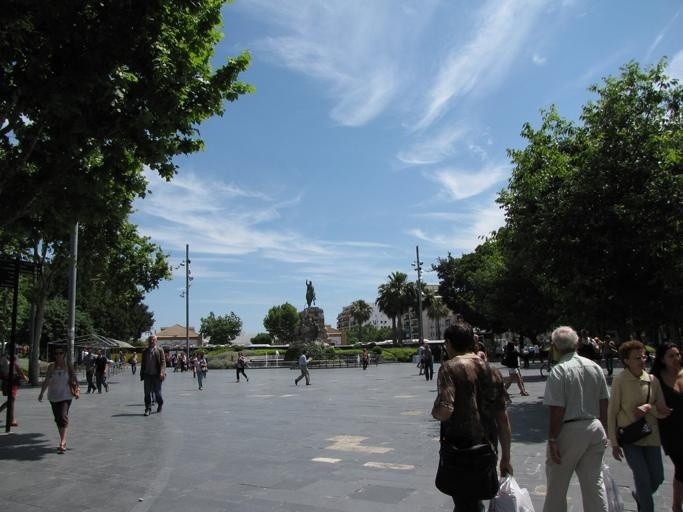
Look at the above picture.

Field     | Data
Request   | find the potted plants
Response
[373,346,383,364]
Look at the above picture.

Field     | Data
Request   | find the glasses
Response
[628,355,647,360]
[54,351,63,355]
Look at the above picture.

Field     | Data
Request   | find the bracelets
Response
[549,438,558,443]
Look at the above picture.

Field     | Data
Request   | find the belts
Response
[560,417,582,425]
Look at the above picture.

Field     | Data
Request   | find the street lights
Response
[179,243,191,359]
[411,244,423,341]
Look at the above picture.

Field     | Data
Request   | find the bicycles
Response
[540,360,556,377]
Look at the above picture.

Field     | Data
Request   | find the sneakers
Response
[157,400,163,412]
[631,490,641,512]
[143,409,150,416]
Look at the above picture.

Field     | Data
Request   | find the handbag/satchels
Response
[436,439,499,501]
[201,359,207,373]
[617,416,652,444]
[66,359,80,397]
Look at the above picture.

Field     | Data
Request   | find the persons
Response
[540,325,609,512]
[305,279,316,307]
[96,350,110,393]
[170,350,209,390]
[607,340,667,512]
[432,321,514,512]
[235,352,249,382]
[295,350,312,386]
[113,349,138,375]
[362,348,370,369]
[81,347,96,394]
[649,340,683,512]
[139,334,168,416]
[0,341,30,426]
[473,332,547,396]
[415,340,446,380]
[37,344,81,453]
[580,329,617,376]
